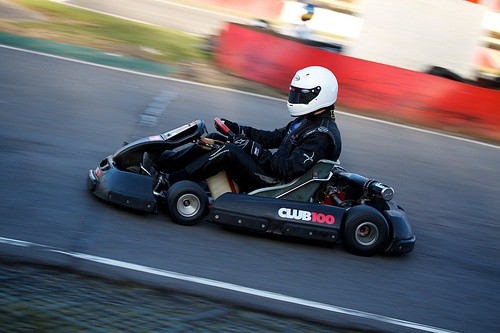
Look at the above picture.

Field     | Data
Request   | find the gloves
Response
[214,118,252,139]
[227,134,272,168]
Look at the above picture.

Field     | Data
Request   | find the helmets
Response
[287,64,338,117]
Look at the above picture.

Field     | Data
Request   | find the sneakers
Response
[142,153,170,200]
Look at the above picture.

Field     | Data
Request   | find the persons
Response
[151,64,344,194]
